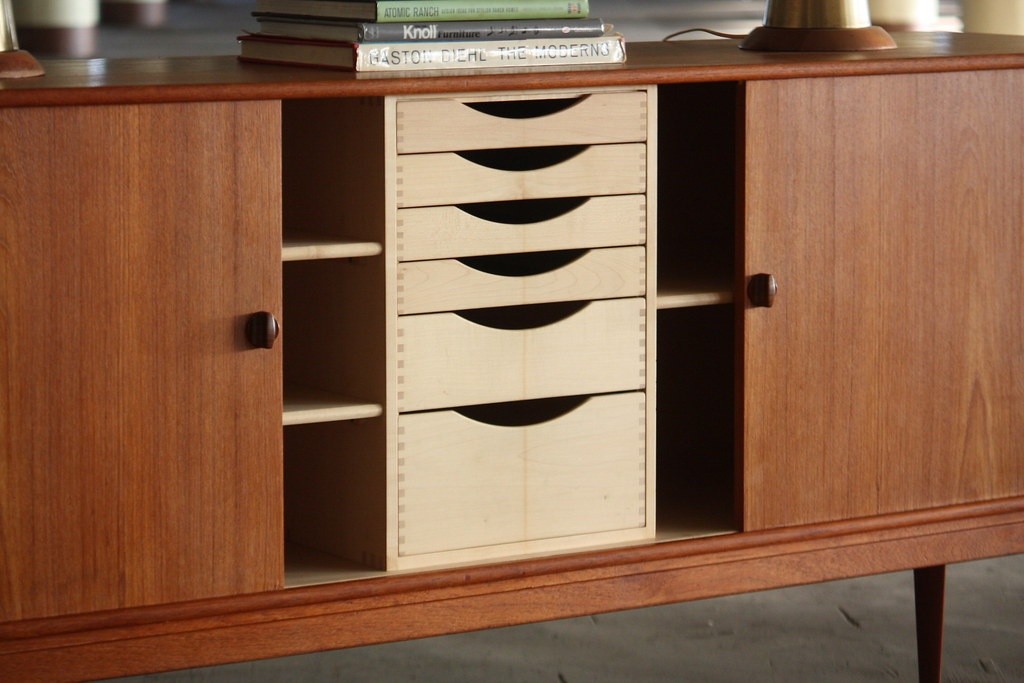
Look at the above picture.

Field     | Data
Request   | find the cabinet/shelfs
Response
[0,32,1024,683]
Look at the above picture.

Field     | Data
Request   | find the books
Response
[236,0,627,73]
